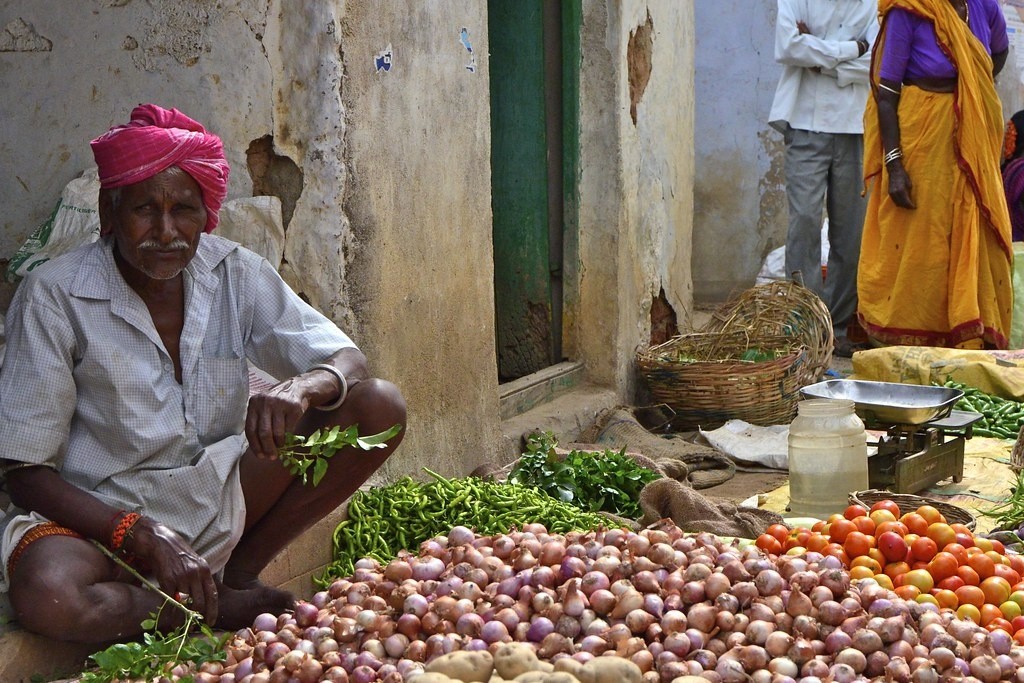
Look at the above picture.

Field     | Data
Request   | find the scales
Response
[797,379,984,495]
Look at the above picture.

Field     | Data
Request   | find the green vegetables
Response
[503,428,664,523]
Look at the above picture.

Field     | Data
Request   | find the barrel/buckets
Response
[786,398,869,521]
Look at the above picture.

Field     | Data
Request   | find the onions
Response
[156,526,1024,683]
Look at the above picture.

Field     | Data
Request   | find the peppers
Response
[312,466,638,598]
[924,375,1024,440]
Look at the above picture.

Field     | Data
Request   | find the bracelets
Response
[304,363,348,412]
[884,146,902,165]
[107,510,141,560]
[878,83,901,95]
[859,41,867,54]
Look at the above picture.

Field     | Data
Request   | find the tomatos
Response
[754,500,1024,647]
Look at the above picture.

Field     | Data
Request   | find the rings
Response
[179,597,193,606]
[175,591,190,602]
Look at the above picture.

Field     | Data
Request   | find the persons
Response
[997,110,1024,241]
[2,102,408,647]
[767,0,881,358]
[856,0,1016,354]
